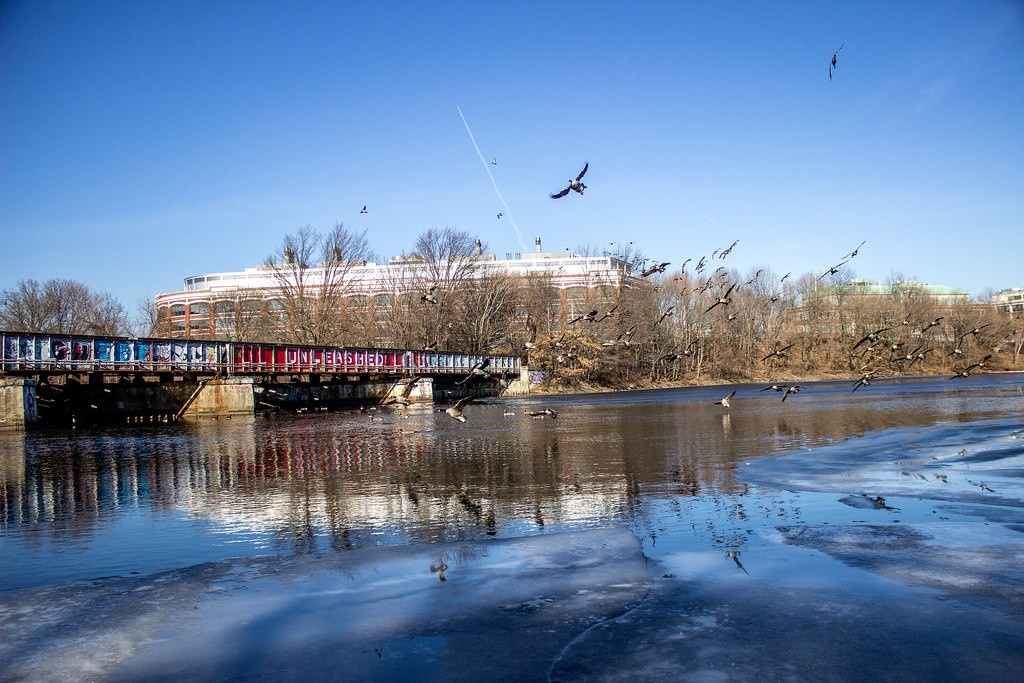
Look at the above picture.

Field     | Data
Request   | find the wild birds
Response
[657,339,800,409]
[829,43,844,80]
[765,240,866,306]
[660,306,675,323]
[504,408,558,419]
[703,282,736,314]
[521,305,637,360]
[550,163,588,199]
[360,157,504,220]
[420,341,439,354]
[673,240,761,294]
[421,285,438,304]
[212,375,423,421]
[437,395,472,423]
[723,311,739,321]
[642,263,671,277]
[852,313,992,393]
[726,451,1004,575]
[491,368,514,395]
[454,358,490,385]
